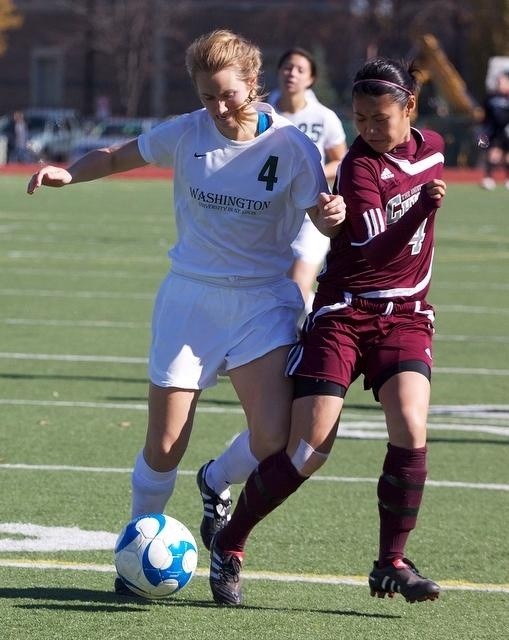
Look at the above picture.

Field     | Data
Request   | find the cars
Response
[0,107,158,166]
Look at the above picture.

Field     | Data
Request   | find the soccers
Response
[113,512,199,600]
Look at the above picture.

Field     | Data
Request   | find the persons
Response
[266,47,348,333]
[482,49,509,195]
[1,109,29,165]
[207,56,449,609]
[24,26,352,596]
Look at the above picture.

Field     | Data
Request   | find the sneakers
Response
[208,530,243,607]
[367,558,440,605]
[113,577,137,597]
[195,458,234,552]
